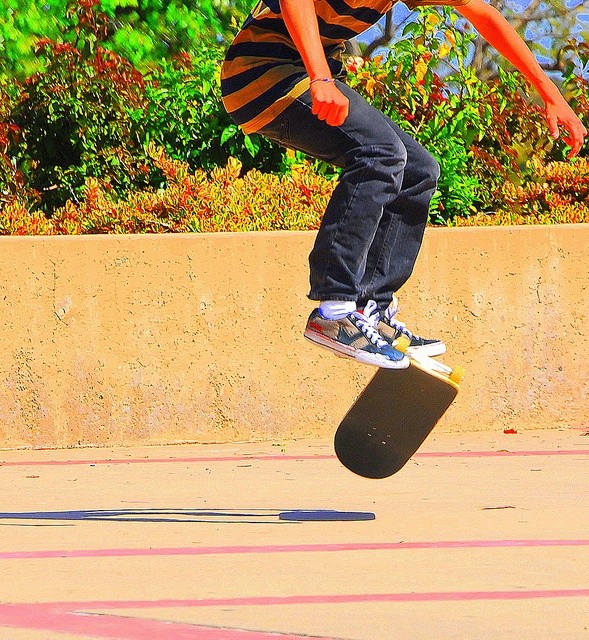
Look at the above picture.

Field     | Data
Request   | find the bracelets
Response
[309,77,336,85]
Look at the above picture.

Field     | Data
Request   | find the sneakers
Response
[303,300,411,369]
[357,294,446,358]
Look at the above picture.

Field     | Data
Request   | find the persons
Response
[221,0,587,370]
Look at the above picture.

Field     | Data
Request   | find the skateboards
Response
[333,336,465,480]
